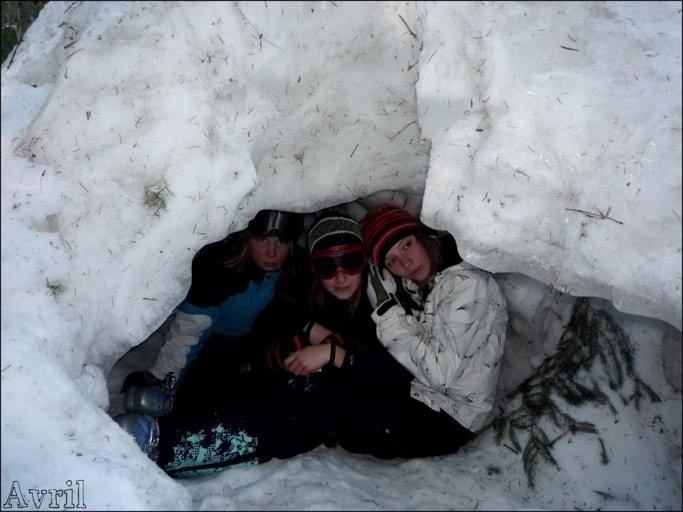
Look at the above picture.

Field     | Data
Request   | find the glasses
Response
[248,209,306,237]
[312,245,366,280]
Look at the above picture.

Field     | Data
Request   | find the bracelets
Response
[305,320,316,337]
[329,339,336,366]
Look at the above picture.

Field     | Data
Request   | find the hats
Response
[304,212,363,257]
[358,201,419,270]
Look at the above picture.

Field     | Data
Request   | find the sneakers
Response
[123,384,175,414]
[111,413,161,456]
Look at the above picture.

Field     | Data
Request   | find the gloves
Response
[362,261,408,324]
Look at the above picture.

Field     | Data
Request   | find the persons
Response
[240,208,412,396]
[112,199,508,476]
[147,207,308,383]
